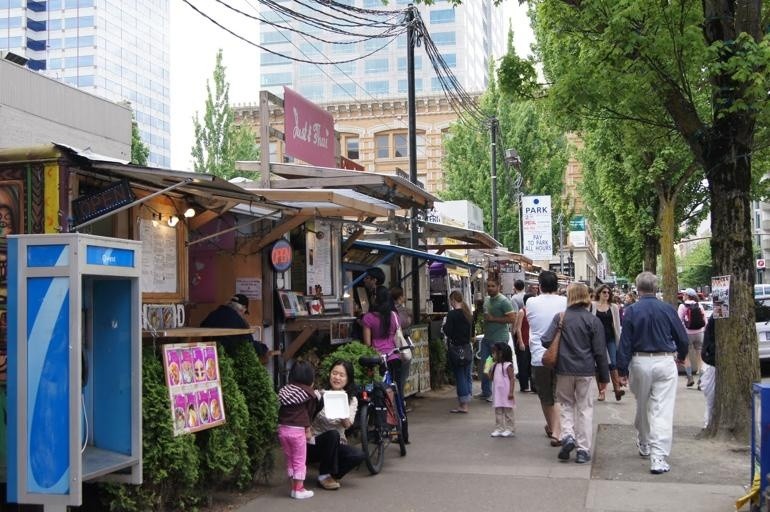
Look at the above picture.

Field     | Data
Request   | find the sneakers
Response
[636,440,650,457]
[490,428,513,437]
[291,488,315,500]
[316,475,340,490]
[648,454,670,473]
[559,437,575,460]
[576,450,590,463]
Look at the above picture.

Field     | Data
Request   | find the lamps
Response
[469,267,482,283]
[300,226,326,242]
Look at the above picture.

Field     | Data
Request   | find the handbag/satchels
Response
[543,330,562,367]
[393,328,413,360]
[447,343,472,367]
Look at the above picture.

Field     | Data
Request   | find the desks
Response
[278,313,358,366]
[140,323,259,342]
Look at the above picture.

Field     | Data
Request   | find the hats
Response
[230,293,252,316]
[681,288,696,296]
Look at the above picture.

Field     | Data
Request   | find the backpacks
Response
[681,301,705,329]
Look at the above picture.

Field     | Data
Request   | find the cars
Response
[675,287,715,318]
[754,295,770,360]
[754,284,770,295]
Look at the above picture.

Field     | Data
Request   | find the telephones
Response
[82,346,88,387]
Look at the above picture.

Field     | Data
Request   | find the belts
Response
[634,351,676,357]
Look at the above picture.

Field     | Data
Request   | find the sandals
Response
[545,423,561,446]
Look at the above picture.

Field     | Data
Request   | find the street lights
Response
[567,243,575,282]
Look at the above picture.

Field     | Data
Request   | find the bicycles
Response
[357,343,417,478]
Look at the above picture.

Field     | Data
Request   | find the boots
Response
[610,369,625,401]
[686,374,694,386]
[594,371,606,401]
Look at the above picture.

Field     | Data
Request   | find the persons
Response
[195,293,269,470]
[363,266,385,293]
[306,359,365,490]
[277,359,324,499]
[356,285,409,445]
[387,285,414,419]
